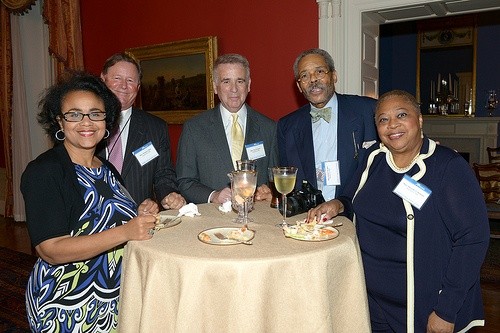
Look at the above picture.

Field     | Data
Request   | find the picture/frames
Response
[123,34,218,125]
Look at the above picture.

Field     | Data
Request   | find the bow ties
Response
[310,107,332,124]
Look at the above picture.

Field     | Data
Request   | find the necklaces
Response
[104,116,132,161]
[388,149,421,171]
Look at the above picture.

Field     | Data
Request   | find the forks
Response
[213,232,253,245]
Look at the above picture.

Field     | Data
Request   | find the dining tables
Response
[116,200,373,333]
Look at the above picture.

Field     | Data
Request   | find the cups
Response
[267,168,282,208]
[236,159,255,172]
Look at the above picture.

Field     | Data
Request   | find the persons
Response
[304,90,491,333]
[177,53,281,205]
[277,49,381,206]
[20,71,159,333]
[96,54,186,211]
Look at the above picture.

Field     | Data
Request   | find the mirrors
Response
[420,44,473,115]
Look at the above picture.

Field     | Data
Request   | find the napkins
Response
[177,202,202,218]
[218,200,233,213]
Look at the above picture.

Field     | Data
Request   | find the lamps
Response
[484,88,499,117]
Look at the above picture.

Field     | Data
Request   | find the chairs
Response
[472,161,500,239]
[487,146,500,166]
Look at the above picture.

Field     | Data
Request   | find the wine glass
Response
[486,90,496,117]
[226,172,254,224]
[272,167,298,227]
[232,171,258,232]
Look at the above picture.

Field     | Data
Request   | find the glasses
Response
[59,111,112,122]
[297,69,331,82]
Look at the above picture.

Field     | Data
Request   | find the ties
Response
[107,123,123,176]
[231,113,244,171]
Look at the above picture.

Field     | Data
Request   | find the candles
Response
[433,80,436,100]
[456,80,457,98]
[453,79,456,97]
[448,73,452,92]
[430,79,432,98]
[437,73,441,93]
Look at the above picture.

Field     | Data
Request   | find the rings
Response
[149,228,154,235]
[181,200,183,202]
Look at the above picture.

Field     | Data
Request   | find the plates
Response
[198,227,254,246]
[284,224,339,241]
[154,215,181,230]
